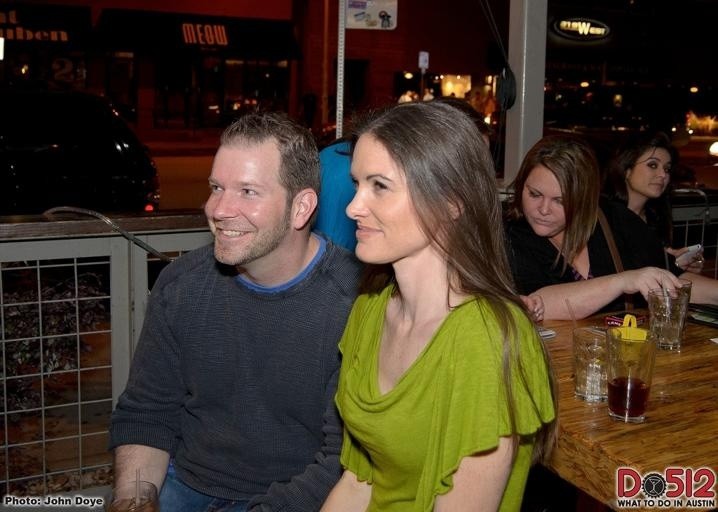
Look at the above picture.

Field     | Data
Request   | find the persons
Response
[514,292,545,323]
[321,105,557,512]
[502,136,682,323]
[108,113,366,512]
[589,128,718,310]
[312,141,357,252]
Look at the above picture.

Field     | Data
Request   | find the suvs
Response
[0,80,162,283]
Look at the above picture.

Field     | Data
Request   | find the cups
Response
[647,289,689,352]
[102,482,159,512]
[676,278,693,302]
[573,327,608,404]
[607,326,658,426]
